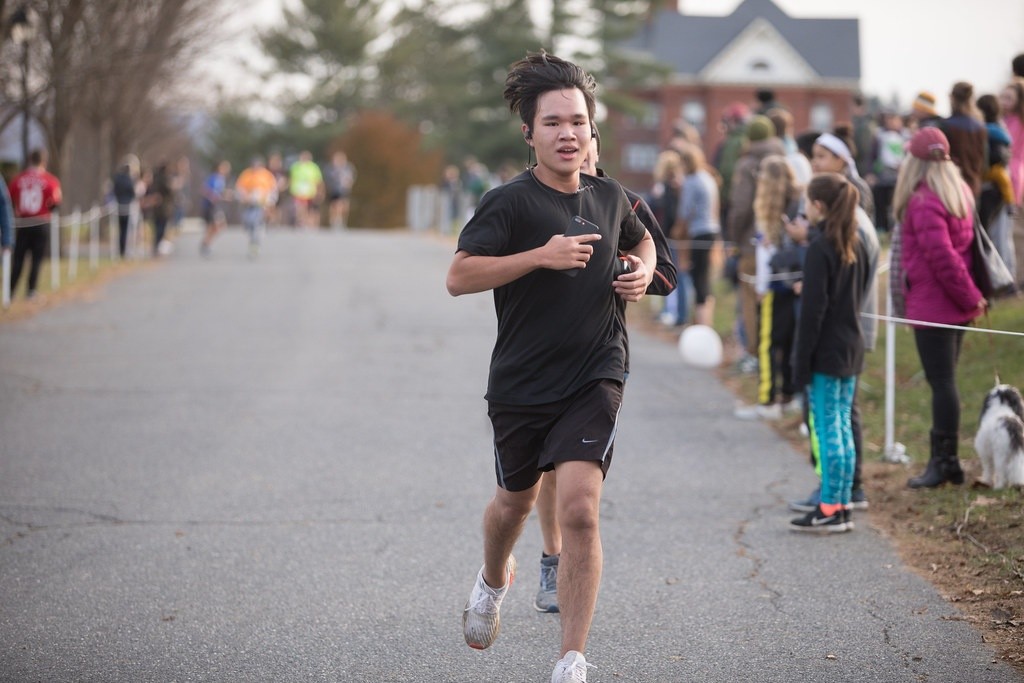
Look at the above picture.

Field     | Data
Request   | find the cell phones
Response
[559,216,599,277]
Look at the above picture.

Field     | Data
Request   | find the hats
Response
[902,126,950,160]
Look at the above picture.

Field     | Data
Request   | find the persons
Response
[849,53,1024,296]
[199,159,232,254]
[642,121,725,341]
[0,148,62,302]
[893,125,989,488]
[790,173,874,533]
[447,47,677,683]
[111,152,187,258]
[714,87,858,418]
[236,149,357,245]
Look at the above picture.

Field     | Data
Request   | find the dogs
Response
[973,382,1024,490]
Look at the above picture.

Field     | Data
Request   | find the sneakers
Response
[462,554,516,649]
[534,555,560,613]
[844,509,854,529]
[789,505,846,532]
[790,487,854,510]
[552,650,596,683]
[851,488,868,507]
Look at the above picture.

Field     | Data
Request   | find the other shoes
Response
[735,404,782,420]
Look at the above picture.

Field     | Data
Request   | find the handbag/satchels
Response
[973,214,1013,298]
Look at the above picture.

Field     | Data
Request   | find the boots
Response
[907,430,963,488]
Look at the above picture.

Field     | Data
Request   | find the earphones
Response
[524,130,532,139]
